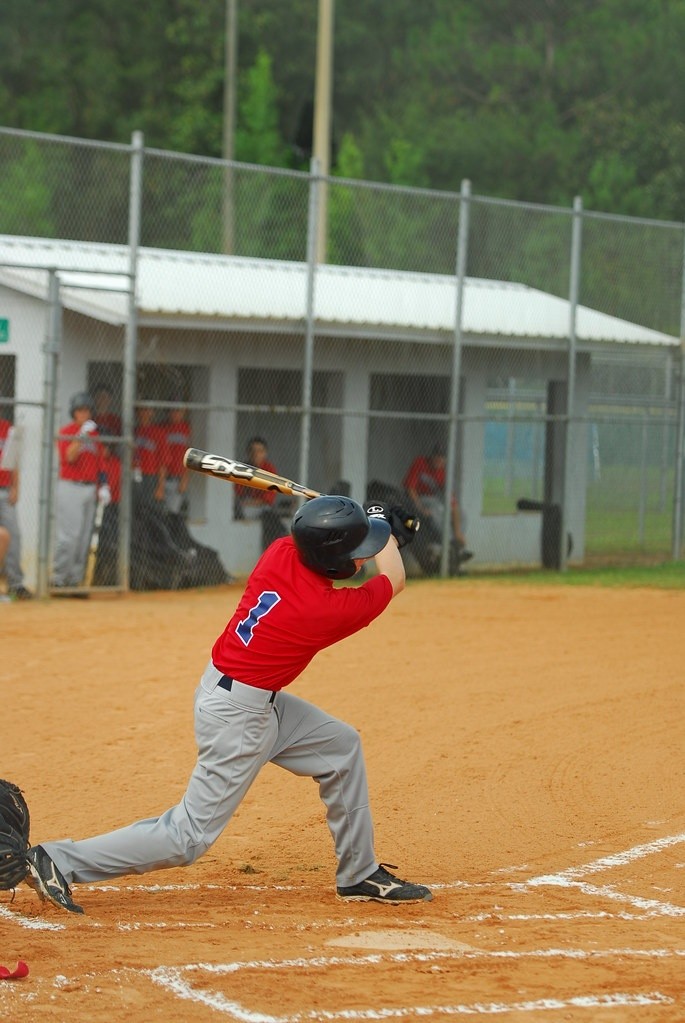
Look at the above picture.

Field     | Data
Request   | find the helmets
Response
[290,496,392,579]
[70,393,96,417]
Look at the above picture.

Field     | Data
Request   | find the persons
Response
[235,436,280,515]
[161,396,191,515]
[49,392,92,598]
[131,397,164,512]
[89,382,123,561]
[0,418,32,598]
[402,440,472,562]
[20,495,431,905]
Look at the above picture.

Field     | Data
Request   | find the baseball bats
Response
[185,446,420,529]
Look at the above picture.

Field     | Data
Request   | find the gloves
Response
[384,502,420,550]
[361,500,392,532]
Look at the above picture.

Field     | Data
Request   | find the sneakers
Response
[336,863,433,904]
[22,844,84,916]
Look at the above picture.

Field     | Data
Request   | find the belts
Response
[205,659,281,706]
